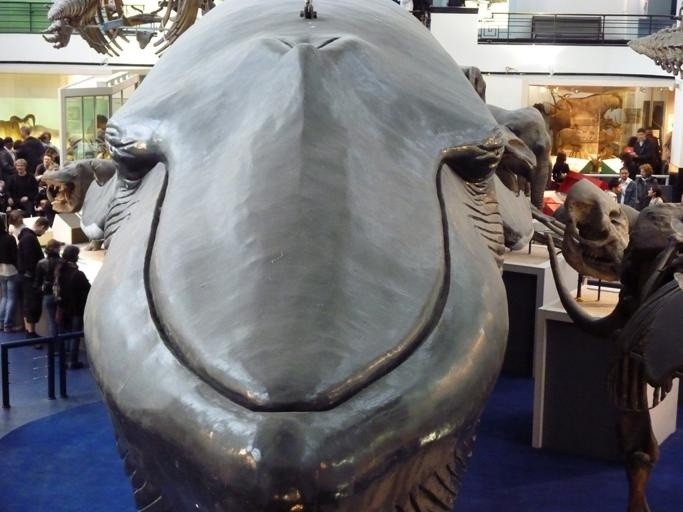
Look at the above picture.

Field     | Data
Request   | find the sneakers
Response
[4,324,22,333]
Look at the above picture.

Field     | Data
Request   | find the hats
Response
[46,239,65,249]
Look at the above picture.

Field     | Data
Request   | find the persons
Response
[0,125,92,371]
[607,127,666,210]
[552,151,569,184]
